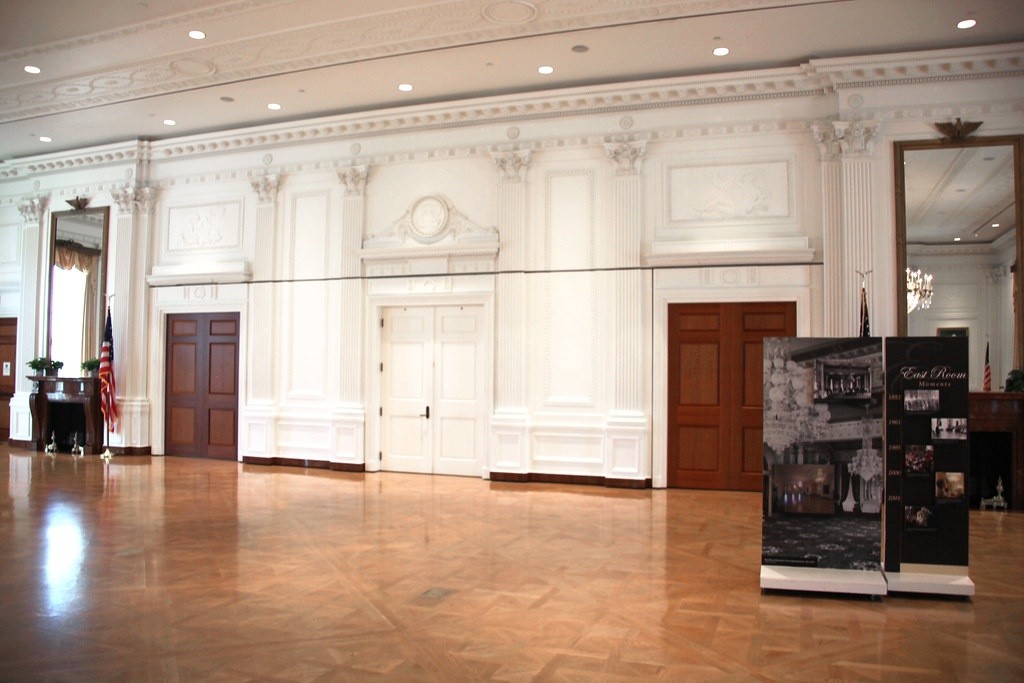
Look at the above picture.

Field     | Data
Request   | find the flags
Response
[98,307,119,435]
[859,287,870,337]
[983,340,992,391]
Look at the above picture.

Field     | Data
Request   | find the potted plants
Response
[26,358,47,377]
[80,358,100,378]
[48,360,64,377]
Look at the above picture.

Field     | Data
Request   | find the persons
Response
[935,478,964,498]
[915,507,931,528]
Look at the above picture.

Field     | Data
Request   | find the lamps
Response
[907,268,933,314]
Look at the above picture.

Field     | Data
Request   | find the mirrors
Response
[45,206,110,380]
[894,134,1023,399]
